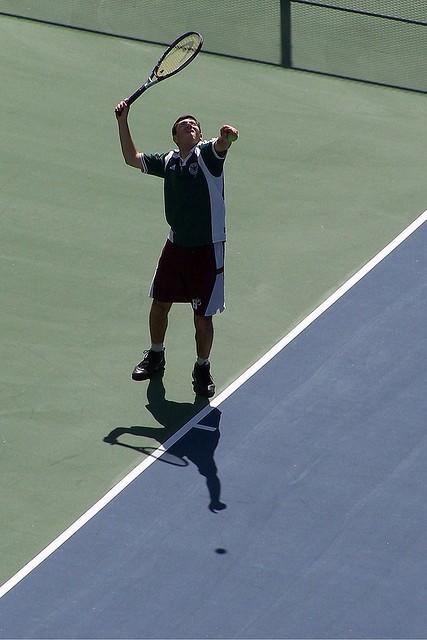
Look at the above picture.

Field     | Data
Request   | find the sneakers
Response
[132,347,165,381]
[192,361,216,397]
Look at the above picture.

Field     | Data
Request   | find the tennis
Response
[227,132,238,141]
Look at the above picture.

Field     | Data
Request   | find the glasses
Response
[174,120,200,126]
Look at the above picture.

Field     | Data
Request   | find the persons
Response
[115,101,239,395]
